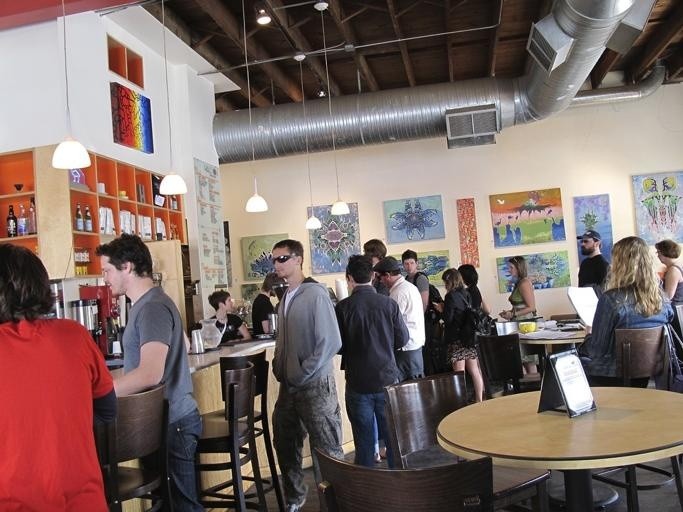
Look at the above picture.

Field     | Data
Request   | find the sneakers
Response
[286,496,307,511]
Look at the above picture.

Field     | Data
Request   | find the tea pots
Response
[197,317,227,349]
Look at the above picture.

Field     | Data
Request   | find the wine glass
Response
[14,184,24,193]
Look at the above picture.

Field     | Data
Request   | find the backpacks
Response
[455,292,483,347]
[406,271,443,322]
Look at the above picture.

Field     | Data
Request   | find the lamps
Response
[313,0,351,223]
[232,0,273,219]
[149,1,190,198]
[293,49,328,235]
[252,7,274,27]
[46,0,95,173]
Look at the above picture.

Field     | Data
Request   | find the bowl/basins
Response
[519,322,536,334]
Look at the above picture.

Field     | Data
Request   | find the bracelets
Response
[512,309,517,318]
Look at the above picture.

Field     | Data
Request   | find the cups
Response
[268,313,278,335]
[191,331,204,353]
[98,182,105,194]
[120,190,126,198]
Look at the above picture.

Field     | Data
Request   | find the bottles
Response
[82,204,92,233]
[18,201,29,236]
[105,317,118,355]
[5,203,17,239]
[29,207,37,235]
[74,201,85,233]
[172,195,179,211]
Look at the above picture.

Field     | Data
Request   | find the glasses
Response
[271,254,293,264]
[377,274,383,281]
[511,257,517,263]
[580,239,592,244]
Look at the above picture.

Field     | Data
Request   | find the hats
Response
[577,229,601,241]
[374,257,400,272]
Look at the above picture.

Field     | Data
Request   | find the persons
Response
[574,231,683,388]
[0,244,122,512]
[94,233,206,512]
[499,256,540,374]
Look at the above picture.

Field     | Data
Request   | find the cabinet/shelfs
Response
[33,142,191,284]
[0,147,41,278]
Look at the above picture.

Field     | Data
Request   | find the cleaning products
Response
[5,205,18,236]
[17,204,28,235]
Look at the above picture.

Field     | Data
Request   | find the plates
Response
[121,196,129,199]
[98,193,109,194]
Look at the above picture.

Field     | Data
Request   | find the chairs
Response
[94,382,174,511]
[575,325,682,511]
[192,360,265,511]
[306,440,495,512]
[473,328,541,398]
[199,348,287,512]
[385,369,555,511]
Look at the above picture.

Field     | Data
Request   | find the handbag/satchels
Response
[664,322,683,393]
